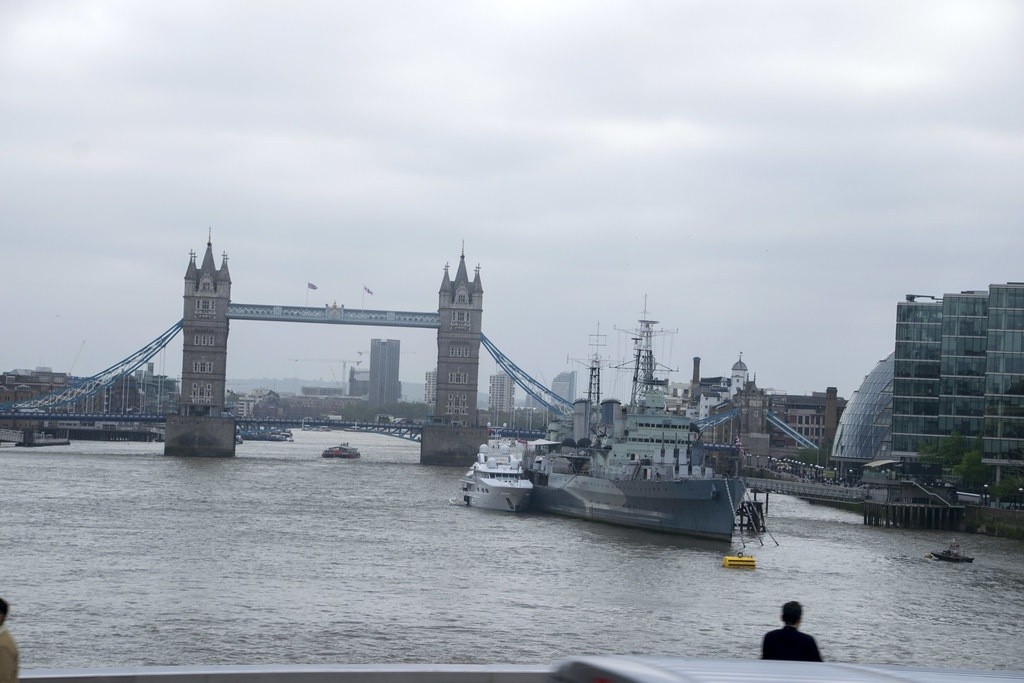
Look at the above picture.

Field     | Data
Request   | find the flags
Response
[308,283,317,290]
[364,286,373,294]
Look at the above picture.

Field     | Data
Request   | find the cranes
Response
[292,357,363,394]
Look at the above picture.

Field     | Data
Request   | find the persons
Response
[762,601,822,661]
[844,482,847,487]
[0,598,18,683]
[654,468,660,480]
[783,466,817,479]
[822,477,843,485]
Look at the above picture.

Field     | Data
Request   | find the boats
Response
[523,294,764,544]
[459,422,535,511]
[932,540,975,564]
[321,442,361,459]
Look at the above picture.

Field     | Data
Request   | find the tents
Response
[527,439,562,453]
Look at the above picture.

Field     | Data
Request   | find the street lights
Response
[849,470,853,488]
[1018,488,1023,510]
[745,453,827,484]
[983,484,989,506]
[833,468,839,487]
